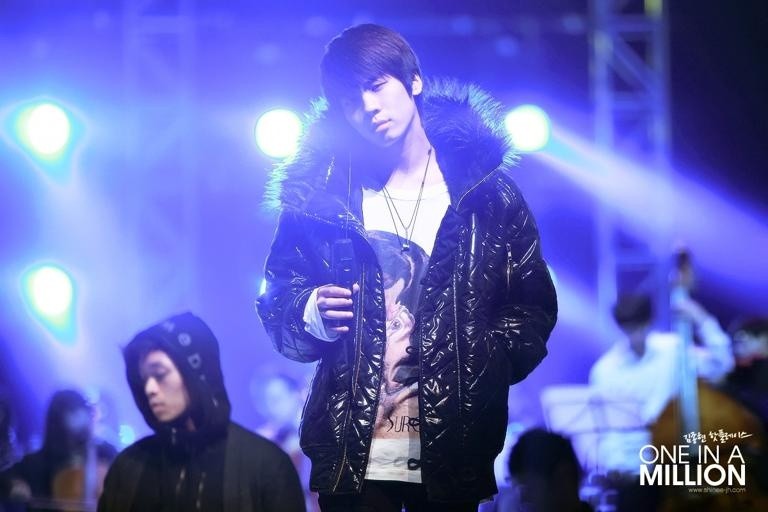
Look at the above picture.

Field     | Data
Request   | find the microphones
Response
[329,237,355,361]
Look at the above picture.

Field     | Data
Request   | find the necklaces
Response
[365,148,437,258]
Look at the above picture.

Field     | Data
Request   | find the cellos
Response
[648,248,767,512]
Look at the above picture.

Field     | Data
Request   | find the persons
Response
[585,283,740,432]
[94,311,307,512]
[713,317,767,424]
[249,23,559,512]
[0,371,768,512]
